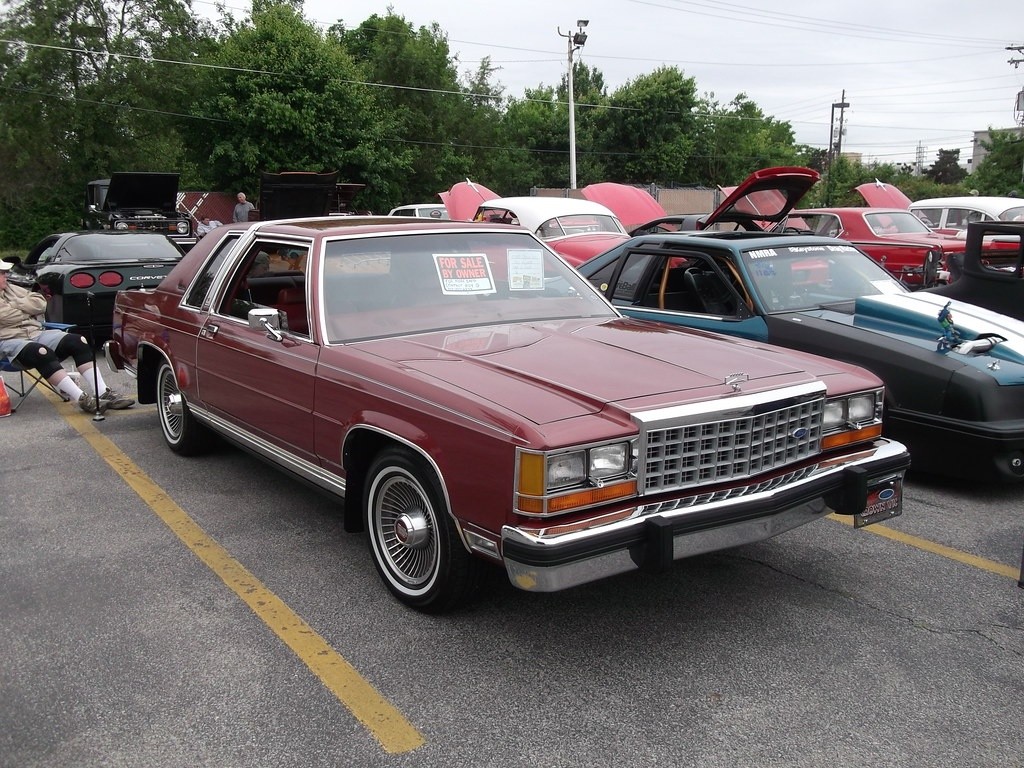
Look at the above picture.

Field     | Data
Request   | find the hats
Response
[0,259,14,270]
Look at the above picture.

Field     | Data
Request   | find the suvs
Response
[83,171,197,253]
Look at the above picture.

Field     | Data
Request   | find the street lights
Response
[558,20,590,190]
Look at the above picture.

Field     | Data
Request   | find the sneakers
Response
[78,391,112,415]
[93,387,135,409]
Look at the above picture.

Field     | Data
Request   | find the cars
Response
[717,185,1020,292]
[1,229,187,342]
[387,203,450,220]
[437,178,687,280]
[581,165,828,286]
[102,216,914,615]
[545,230,1024,481]
[855,178,1024,237]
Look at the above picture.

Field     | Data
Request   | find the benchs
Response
[664,267,737,316]
[236,276,309,336]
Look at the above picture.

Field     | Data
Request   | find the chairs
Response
[0,322,77,414]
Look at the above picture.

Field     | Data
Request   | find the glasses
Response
[0,270,9,274]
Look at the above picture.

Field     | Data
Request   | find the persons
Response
[1008,191,1018,198]
[232,191,255,223]
[967,189,979,197]
[940,300,968,348]
[196,215,223,238]
[0,257,135,413]
